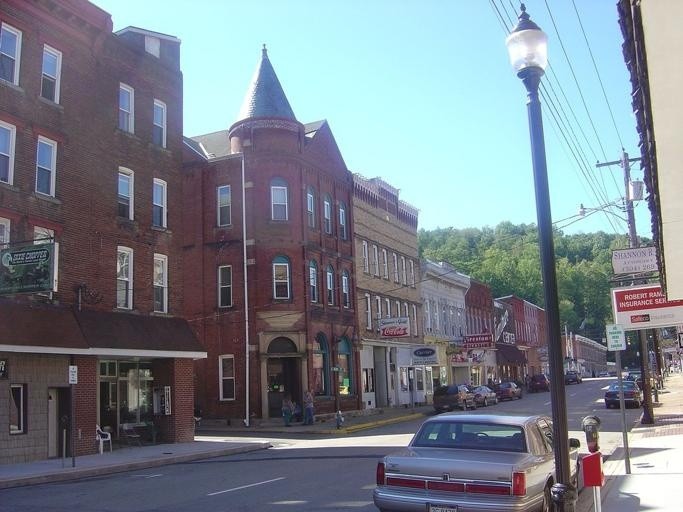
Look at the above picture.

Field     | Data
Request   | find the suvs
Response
[530,374,550,392]
[432,384,480,412]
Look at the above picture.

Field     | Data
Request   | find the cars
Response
[494,381,522,401]
[474,385,499,407]
[604,369,656,409]
[373,409,582,512]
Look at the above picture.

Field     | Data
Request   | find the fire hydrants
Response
[335,410,345,429]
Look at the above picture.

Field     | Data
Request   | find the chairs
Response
[96,424,113,455]
[121,423,142,450]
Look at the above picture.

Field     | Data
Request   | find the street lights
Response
[508,1,575,512]
[579,201,639,249]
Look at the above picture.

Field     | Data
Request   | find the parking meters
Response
[580,414,607,512]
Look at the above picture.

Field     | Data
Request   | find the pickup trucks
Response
[565,370,582,384]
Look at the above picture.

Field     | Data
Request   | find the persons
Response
[278,387,314,427]
[58,415,70,442]
